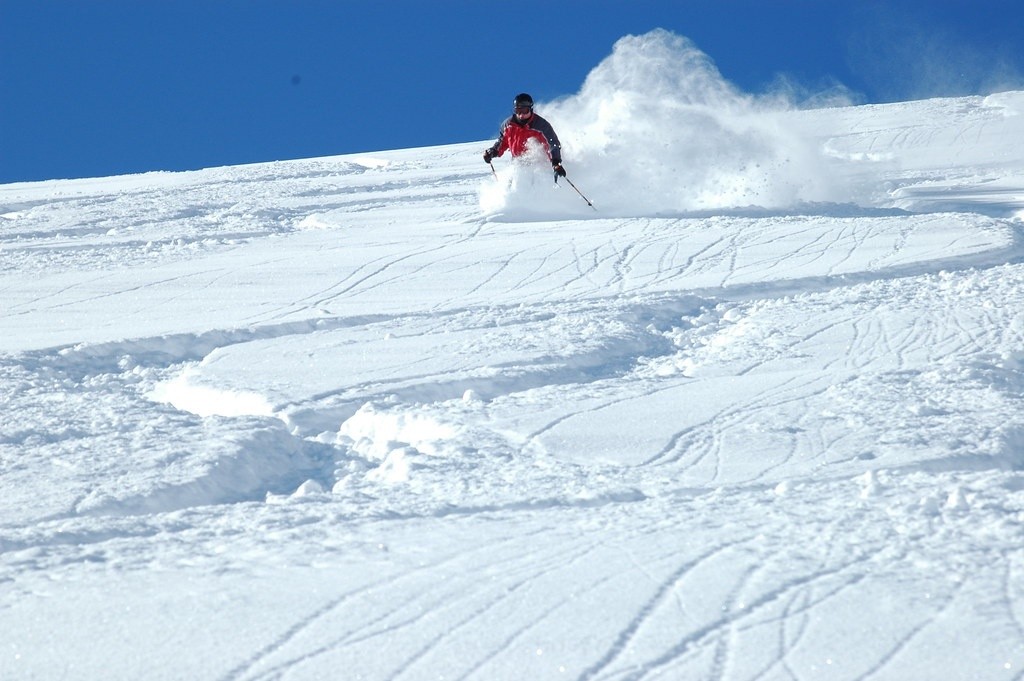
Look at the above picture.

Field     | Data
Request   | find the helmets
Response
[513,93,533,106]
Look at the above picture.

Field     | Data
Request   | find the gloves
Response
[483,152,492,164]
[555,166,566,177]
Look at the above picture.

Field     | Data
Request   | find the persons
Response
[483,93,566,184]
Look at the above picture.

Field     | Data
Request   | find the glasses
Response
[514,108,531,115]
